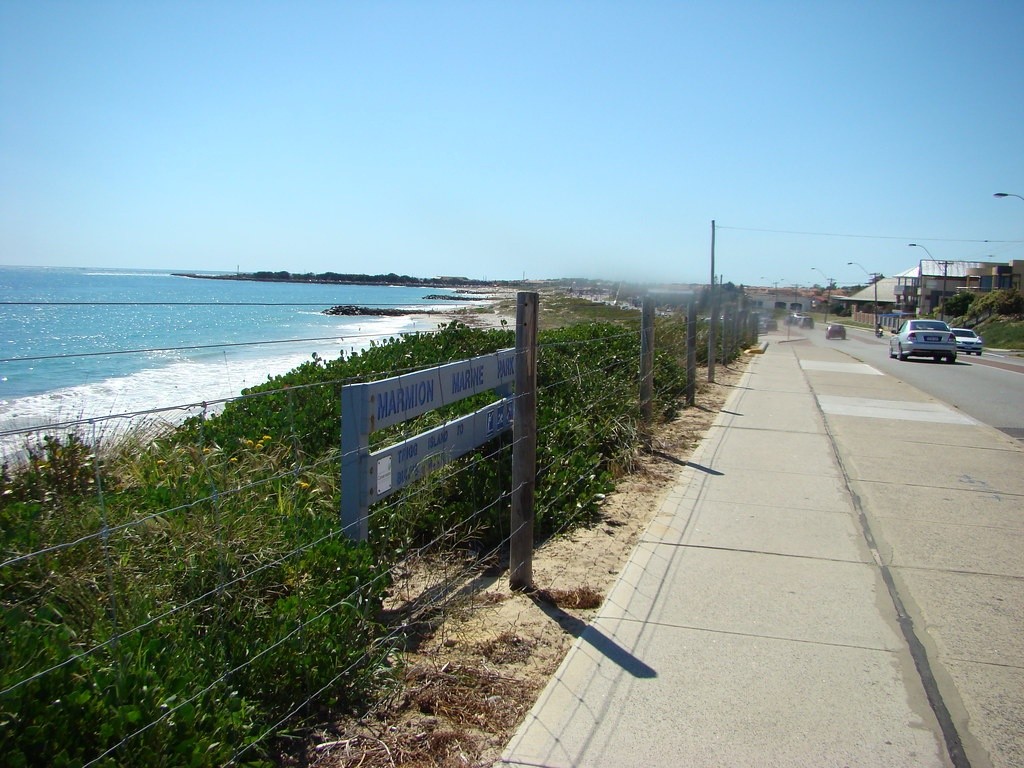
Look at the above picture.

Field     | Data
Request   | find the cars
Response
[889,319,957,364]
[825,323,846,340]
[949,328,983,356]
[784,311,815,329]
[703,310,778,335]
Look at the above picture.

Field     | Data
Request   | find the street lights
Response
[908,243,954,322]
[848,262,879,325]
[810,267,836,322]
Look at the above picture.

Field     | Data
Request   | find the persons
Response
[875,322,883,335]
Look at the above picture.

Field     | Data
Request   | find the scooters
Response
[875,328,884,339]
